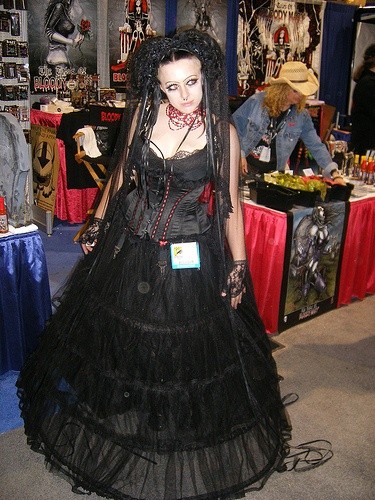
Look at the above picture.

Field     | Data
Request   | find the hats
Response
[269,62,319,96]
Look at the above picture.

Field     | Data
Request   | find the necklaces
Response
[165,104,207,131]
[267,118,288,145]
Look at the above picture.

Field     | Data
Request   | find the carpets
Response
[0,224,86,433]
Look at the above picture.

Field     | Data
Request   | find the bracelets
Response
[333,177,343,181]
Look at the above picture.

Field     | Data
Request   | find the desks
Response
[31,99,131,223]
[237,169,375,336]
[0,220,52,376]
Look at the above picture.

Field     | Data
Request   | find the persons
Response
[15,29,291,499]
[347,44,375,163]
[231,61,347,187]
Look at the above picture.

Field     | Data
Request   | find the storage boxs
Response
[248,177,354,212]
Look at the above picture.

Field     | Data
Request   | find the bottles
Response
[0,190,9,233]
